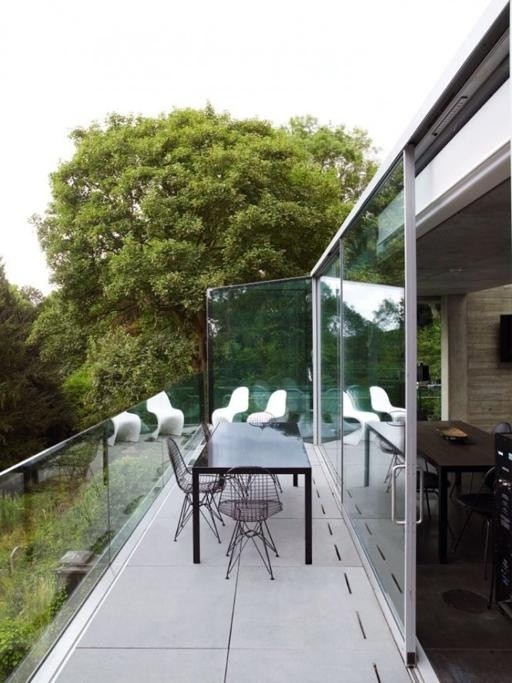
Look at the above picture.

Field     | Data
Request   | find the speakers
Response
[491,432,512,627]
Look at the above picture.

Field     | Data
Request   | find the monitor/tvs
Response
[499,314,512,363]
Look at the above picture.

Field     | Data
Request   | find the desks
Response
[417,419,498,565]
[363,419,405,487]
[191,421,313,566]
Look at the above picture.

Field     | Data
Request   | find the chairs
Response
[166,438,226,545]
[453,466,497,581]
[417,459,456,550]
[449,421,512,499]
[247,389,288,426]
[211,385,251,426]
[379,410,407,495]
[342,392,381,446]
[201,421,283,496]
[107,411,142,447]
[144,391,185,439]
[369,385,406,423]
[218,464,284,581]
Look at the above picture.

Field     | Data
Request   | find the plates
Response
[435,426,468,441]
